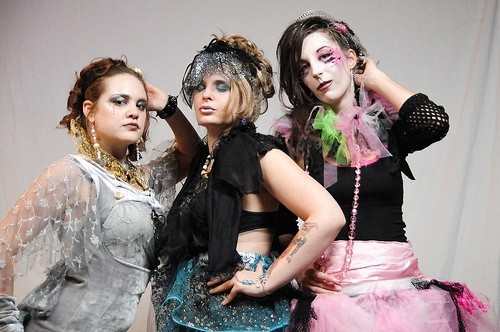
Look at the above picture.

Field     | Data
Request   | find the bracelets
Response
[259,270,272,295]
[157,95,177,119]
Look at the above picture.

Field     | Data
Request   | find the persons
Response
[267,10,500,332]
[144,30,347,332]
[0,55,202,332]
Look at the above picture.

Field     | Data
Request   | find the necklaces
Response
[78,140,140,186]
[304,133,361,285]
[200,153,216,180]
